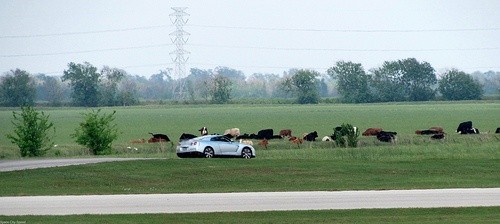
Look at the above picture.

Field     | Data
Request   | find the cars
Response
[175,135,256,159]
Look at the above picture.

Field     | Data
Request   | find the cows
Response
[130,125,400,145]
[414,121,499,140]
